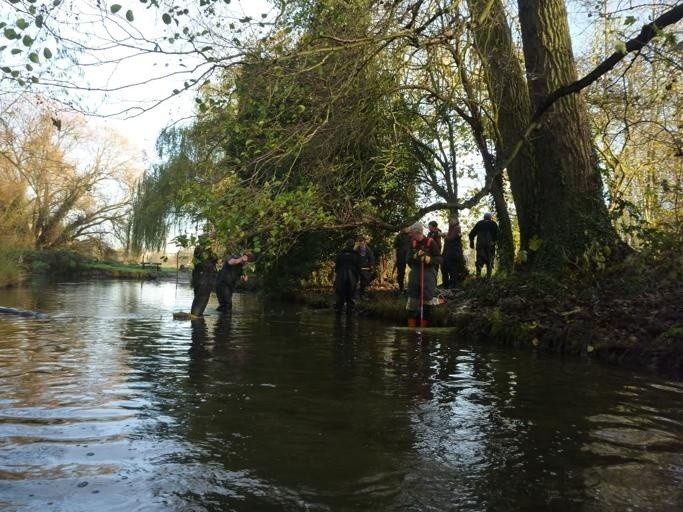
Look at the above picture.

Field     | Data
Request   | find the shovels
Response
[407,254,428,328]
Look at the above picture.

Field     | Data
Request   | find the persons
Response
[427,221,441,288]
[393,225,415,295]
[216,250,249,314]
[333,238,360,315]
[190,233,219,316]
[353,238,375,301]
[405,223,444,326]
[440,215,461,287]
[468,213,500,279]
